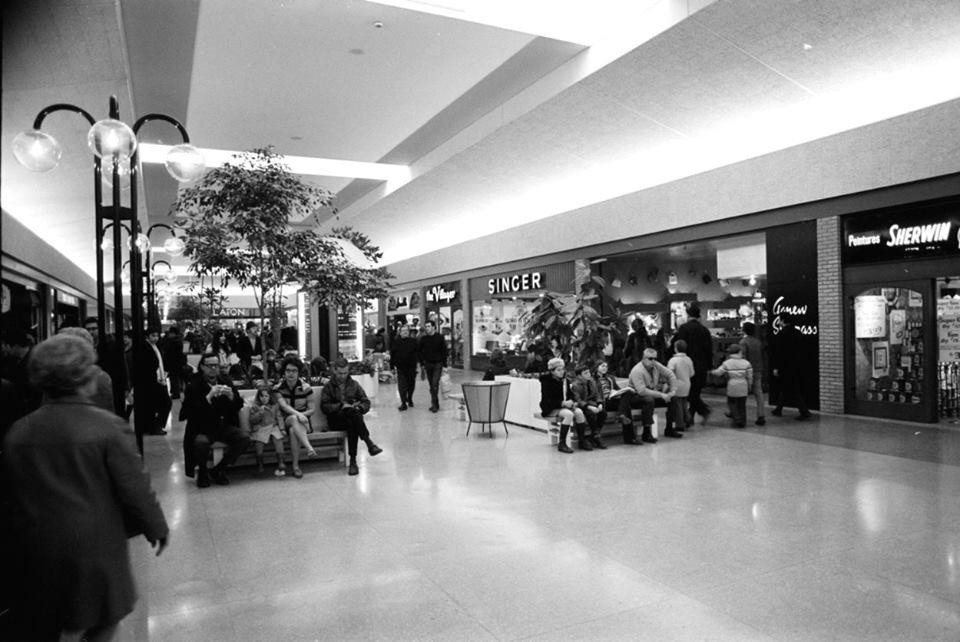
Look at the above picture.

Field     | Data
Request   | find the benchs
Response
[208,432,352,468]
[533,407,668,448]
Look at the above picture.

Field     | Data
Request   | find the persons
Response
[624,319,652,378]
[178,351,245,489]
[249,357,318,479]
[665,305,814,429]
[318,358,382,475]
[540,354,644,452]
[373,316,448,412]
[630,346,684,441]
[109,319,329,396]
[0,314,169,642]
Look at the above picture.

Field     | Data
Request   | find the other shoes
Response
[150,428,167,435]
[642,433,659,444]
[210,467,230,485]
[197,470,210,487]
[723,410,812,428]
[665,407,714,439]
[590,434,608,449]
[368,445,383,456]
[348,463,360,475]
[399,404,408,411]
[626,438,643,445]
[407,401,414,407]
[293,468,304,478]
[429,405,441,412]
[308,450,318,458]
[614,414,632,425]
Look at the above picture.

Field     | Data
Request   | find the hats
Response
[547,357,570,368]
[685,305,702,317]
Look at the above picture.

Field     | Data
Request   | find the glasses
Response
[202,362,221,369]
[285,368,299,373]
[648,358,658,361]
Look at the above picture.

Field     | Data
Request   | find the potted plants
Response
[494,257,653,435]
[166,144,397,435]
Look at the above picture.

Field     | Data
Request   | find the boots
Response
[575,423,594,451]
[276,452,288,471]
[557,424,572,454]
[256,454,267,476]
